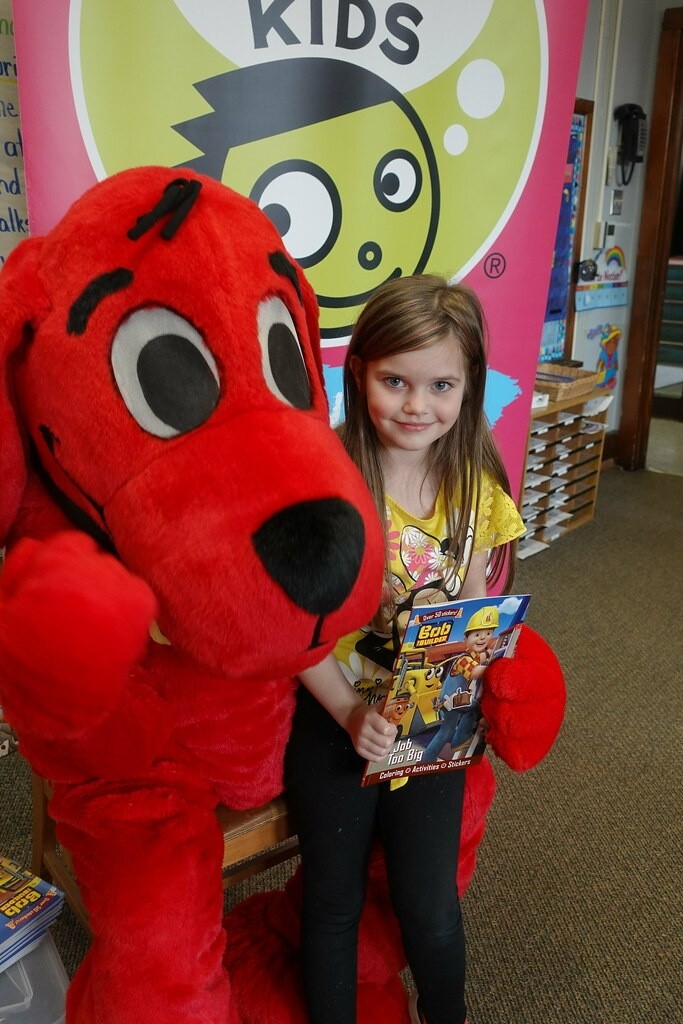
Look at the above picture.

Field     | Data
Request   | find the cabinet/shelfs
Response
[511,386,614,560]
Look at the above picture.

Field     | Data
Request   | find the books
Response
[362,593,531,788]
[0,857,66,975]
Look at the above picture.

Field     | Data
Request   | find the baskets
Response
[534,363,599,402]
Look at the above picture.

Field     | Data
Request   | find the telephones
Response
[613,103,648,166]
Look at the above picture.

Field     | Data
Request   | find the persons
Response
[287,275,528,1023]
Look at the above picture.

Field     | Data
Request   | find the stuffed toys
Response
[0,168,567,1024]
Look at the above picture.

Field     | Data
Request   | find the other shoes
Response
[408,988,468,1024]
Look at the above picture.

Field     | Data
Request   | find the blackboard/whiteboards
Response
[532,92,595,367]
